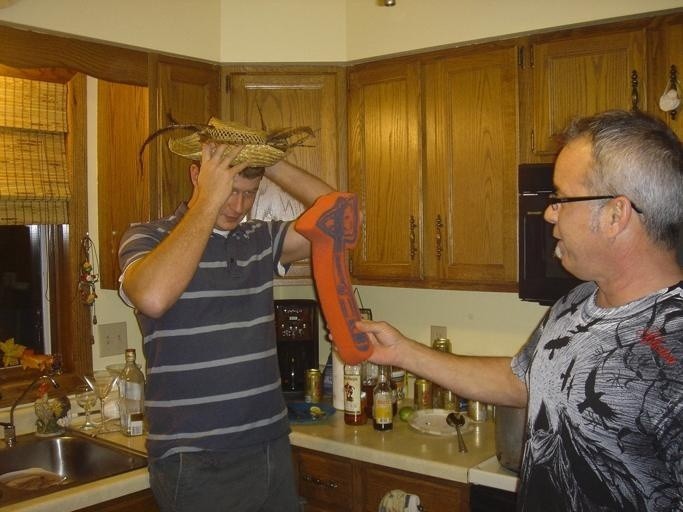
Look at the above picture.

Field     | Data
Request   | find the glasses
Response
[549,192,644,216]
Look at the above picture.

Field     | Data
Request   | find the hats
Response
[167,115,291,168]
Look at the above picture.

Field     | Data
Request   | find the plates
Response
[408,407,471,436]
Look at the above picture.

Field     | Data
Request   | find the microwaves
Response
[518,162,584,307]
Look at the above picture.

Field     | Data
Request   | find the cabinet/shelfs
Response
[344,31,522,293]
[291,445,360,512]
[218,62,345,287]
[96,48,218,290]
[360,461,472,512]
[525,19,682,164]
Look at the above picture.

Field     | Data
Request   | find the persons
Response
[325,108,682,511]
[117,117,363,512]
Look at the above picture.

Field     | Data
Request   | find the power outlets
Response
[98,322,128,358]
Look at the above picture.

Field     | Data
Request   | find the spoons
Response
[446,411,468,452]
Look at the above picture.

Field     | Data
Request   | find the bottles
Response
[343,359,406,433]
[118,349,144,437]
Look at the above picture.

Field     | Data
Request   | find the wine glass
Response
[74,362,141,434]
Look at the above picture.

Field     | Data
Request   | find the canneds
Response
[414,379,433,412]
[469,401,486,423]
[303,369,322,404]
[444,390,457,410]
[434,338,449,352]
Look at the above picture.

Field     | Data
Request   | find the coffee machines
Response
[272,277,330,403]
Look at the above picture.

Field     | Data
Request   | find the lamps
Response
[384,0,395,6]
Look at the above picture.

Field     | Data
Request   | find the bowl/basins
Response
[493,404,528,472]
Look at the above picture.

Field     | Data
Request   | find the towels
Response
[378,489,421,512]
